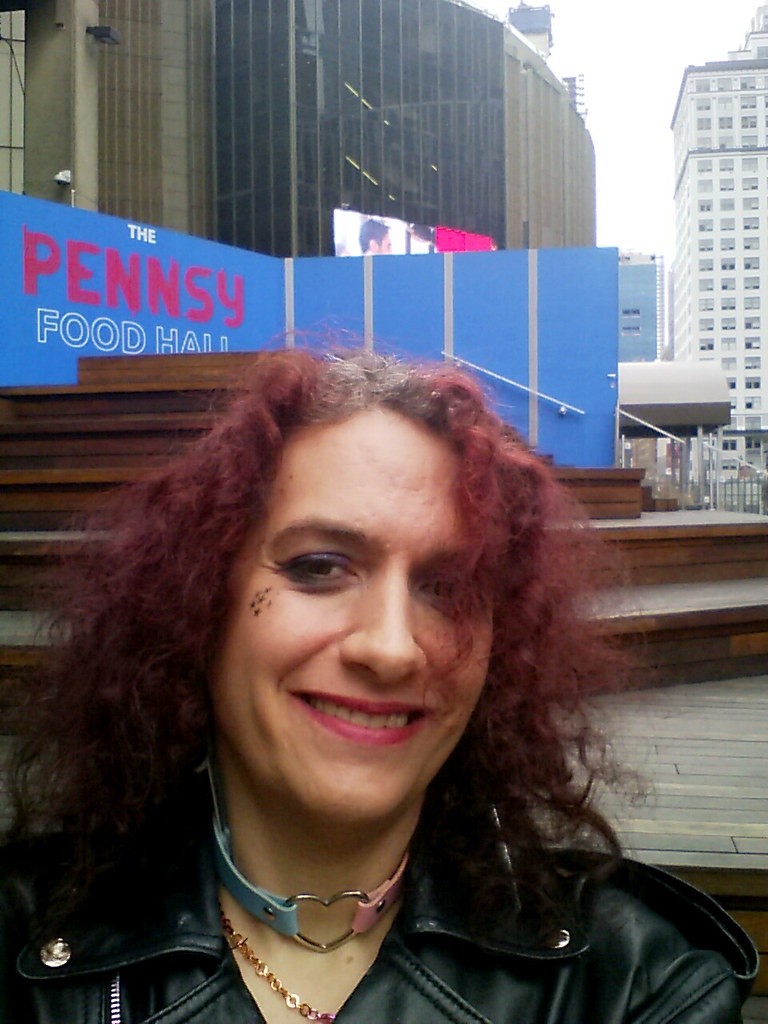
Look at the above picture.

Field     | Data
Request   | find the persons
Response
[359,219,393,255]
[0,345,768,1024]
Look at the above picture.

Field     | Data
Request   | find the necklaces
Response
[218,903,337,1024]
[213,815,407,952]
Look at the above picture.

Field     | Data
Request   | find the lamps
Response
[86,25,120,46]
